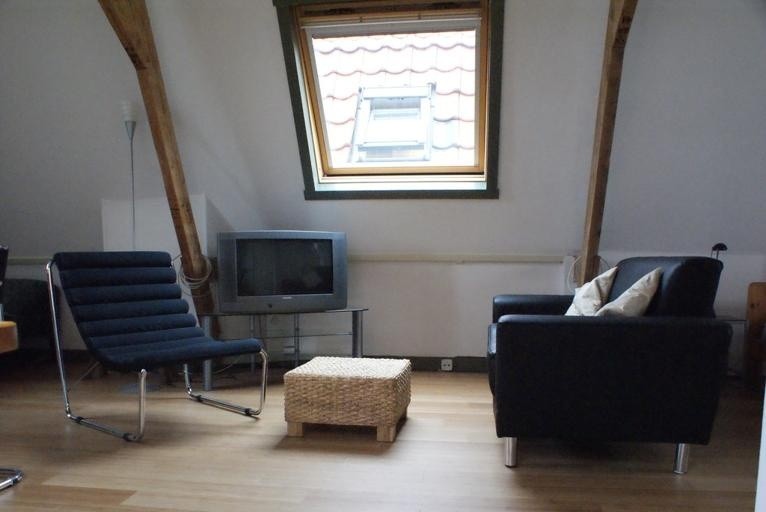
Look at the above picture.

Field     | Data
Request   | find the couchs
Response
[486,254,725,474]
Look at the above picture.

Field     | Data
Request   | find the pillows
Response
[596,267,661,318]
[564,268,618,319]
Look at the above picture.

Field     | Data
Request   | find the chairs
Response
[45,250,268,442]
[6,275,61,357]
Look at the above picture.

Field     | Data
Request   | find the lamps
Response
[117,98,140,251]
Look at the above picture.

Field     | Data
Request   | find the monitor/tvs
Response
[217,230,347,315]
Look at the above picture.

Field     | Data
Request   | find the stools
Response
[0,320,25,490]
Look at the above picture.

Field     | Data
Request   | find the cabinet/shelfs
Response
[197,306,368,389]
